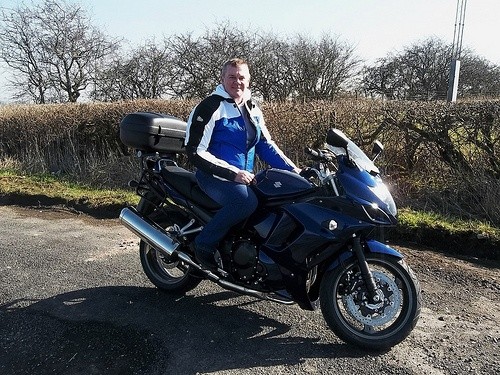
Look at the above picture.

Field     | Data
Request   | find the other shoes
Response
[190,239,219,271]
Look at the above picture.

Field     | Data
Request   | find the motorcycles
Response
[118,112,423,352]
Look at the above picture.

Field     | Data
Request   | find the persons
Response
[185,58,320,271]
